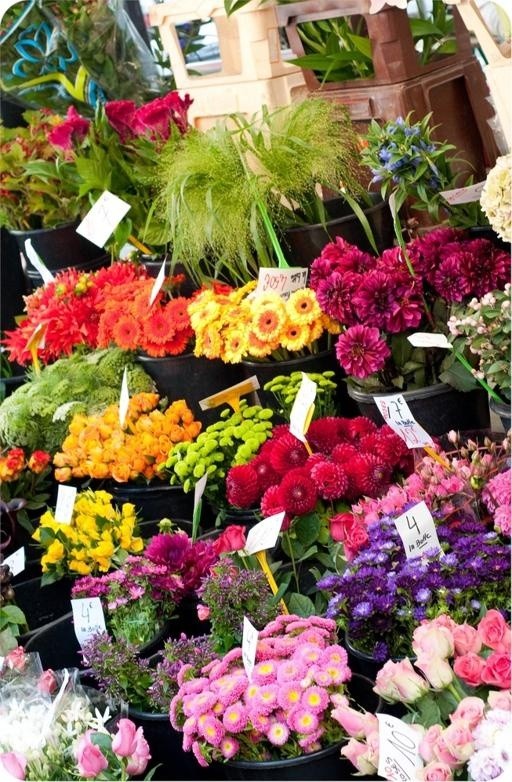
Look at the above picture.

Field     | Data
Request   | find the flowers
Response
[1,0,511,780]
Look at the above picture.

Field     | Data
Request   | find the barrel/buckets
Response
[1,215,512,781]
